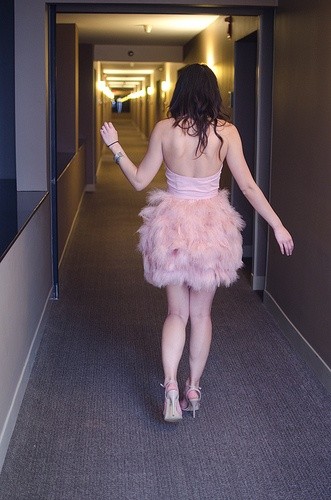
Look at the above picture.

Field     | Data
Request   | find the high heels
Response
[159,379,185,425]
[182,386,203,421]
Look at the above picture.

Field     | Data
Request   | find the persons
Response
[101,63,295,422]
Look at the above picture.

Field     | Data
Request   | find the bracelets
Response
[108,141,119,148]
[114,152,123,165]
